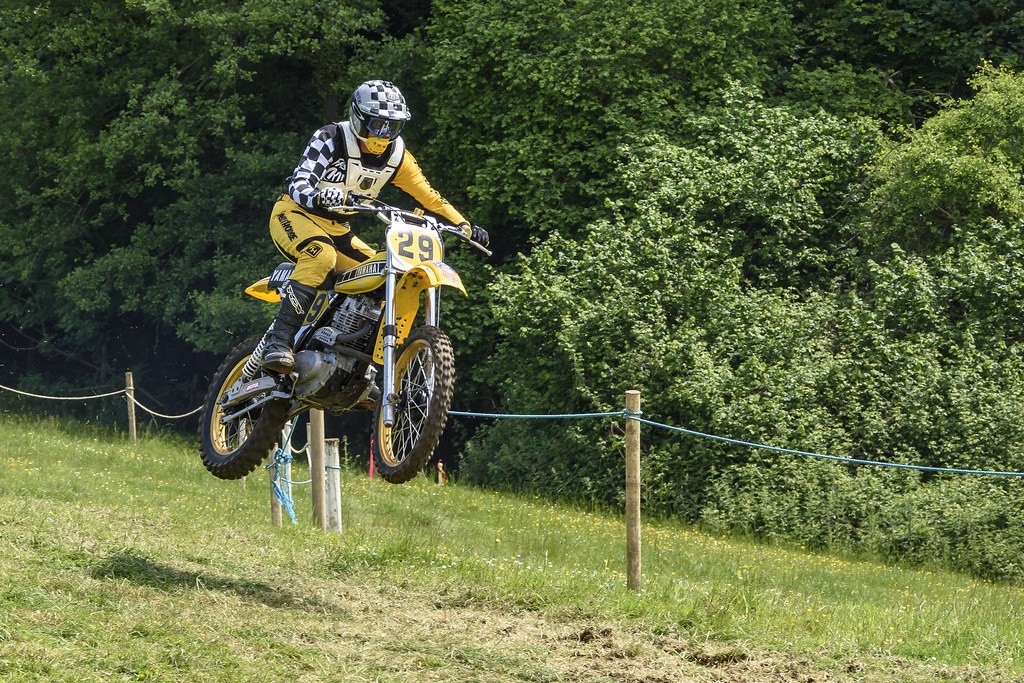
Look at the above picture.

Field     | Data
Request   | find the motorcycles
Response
[197,186,494,487]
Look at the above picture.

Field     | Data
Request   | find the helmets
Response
[349,79,411,134]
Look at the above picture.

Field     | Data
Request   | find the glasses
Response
[366,117,402,139]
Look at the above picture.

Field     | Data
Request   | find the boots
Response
[262,279,318,375]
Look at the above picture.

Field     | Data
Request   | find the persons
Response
[259,80,489,374]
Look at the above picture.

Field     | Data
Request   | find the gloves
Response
[471,225,489,247]
[320,187,344,206]
